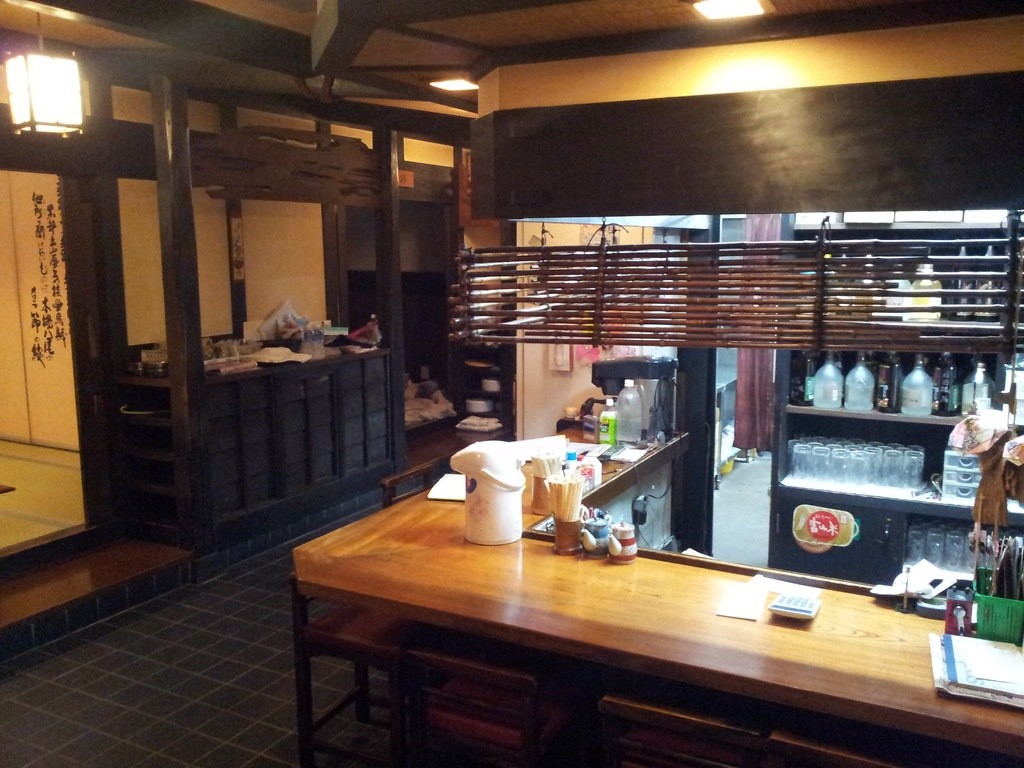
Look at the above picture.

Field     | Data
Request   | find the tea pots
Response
[580,517,611,558]
[607,518,637,564]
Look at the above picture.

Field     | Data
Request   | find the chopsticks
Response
[548,475,584,521]
[531,454,561,478]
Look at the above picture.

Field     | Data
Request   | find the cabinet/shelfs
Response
[116,341,393,551]
[767,492,904,590]
[776,318,1003,499]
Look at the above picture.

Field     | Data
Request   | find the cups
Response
[787,435,925,492]
[907,518,995,574]
[534,476,552,515]
[553,516,582,554]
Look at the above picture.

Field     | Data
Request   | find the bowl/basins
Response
[130,360,167,379]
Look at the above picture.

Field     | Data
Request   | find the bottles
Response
[617,379,642,442]
[304,324,314,356]
[791,349,996,416]
[314,324,324,357]
[599,399,617,445]
[801,234,1002,322]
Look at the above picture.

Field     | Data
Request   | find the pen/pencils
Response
[603,468,619,475]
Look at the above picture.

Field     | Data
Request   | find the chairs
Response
[289,581,898,767]
[378,448,467,509]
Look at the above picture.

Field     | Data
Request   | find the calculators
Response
[767,593,821,619]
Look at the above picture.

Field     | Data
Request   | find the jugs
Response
[449,440,527,546]
